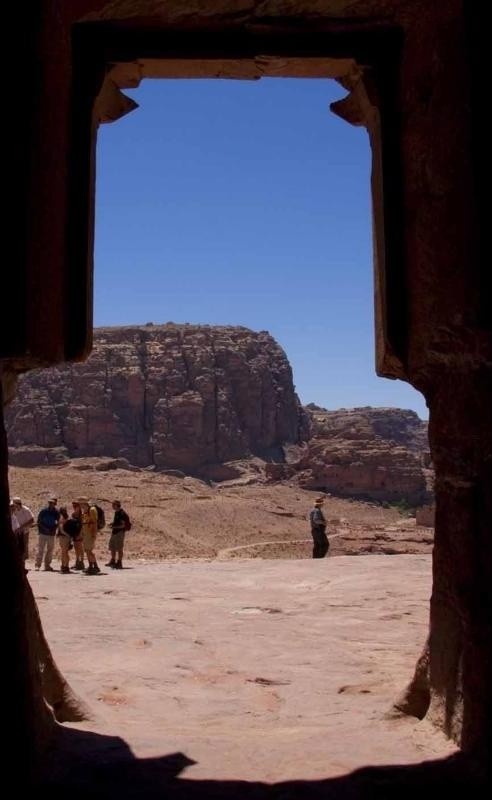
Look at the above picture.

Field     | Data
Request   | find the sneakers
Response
[35,558,122,575]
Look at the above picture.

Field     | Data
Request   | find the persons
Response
[8,493,128,576]
[309,495,330,558]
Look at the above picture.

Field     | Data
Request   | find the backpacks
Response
[120,511,132,532]
[94,505,106,530]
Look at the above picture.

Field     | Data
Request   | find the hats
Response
[12,495,121,506]
[312,497,326,505]
